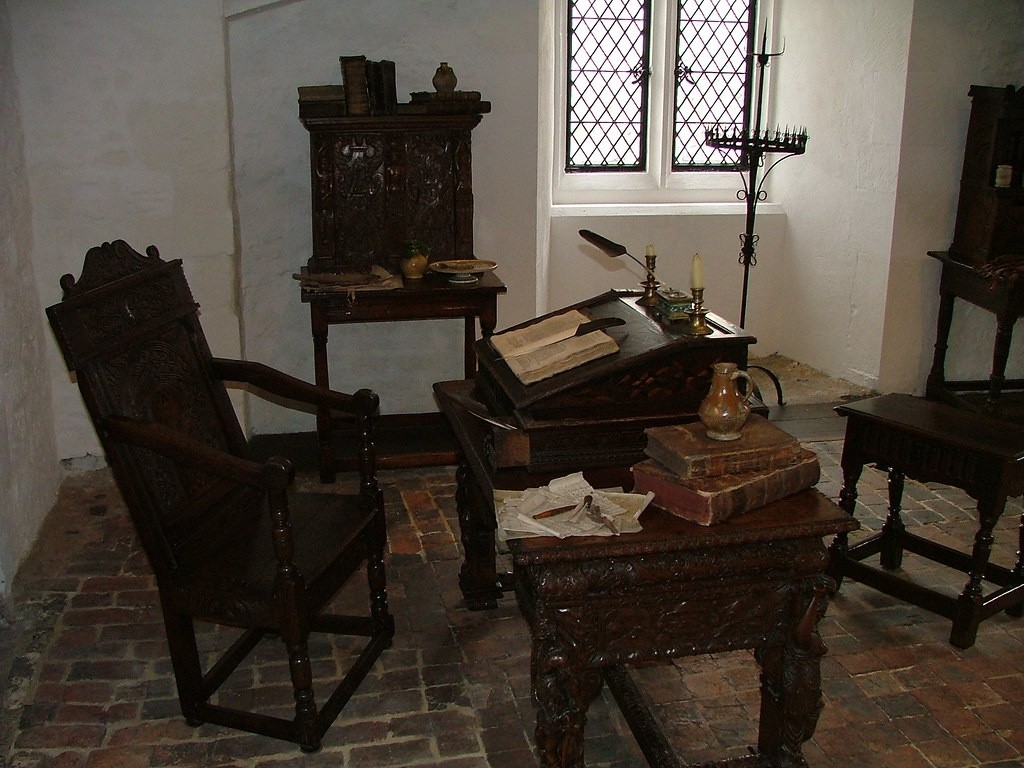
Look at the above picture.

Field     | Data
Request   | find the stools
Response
[820,393,1024,650]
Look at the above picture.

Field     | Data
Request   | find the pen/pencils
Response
[533,505,577,520]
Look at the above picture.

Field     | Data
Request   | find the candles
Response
[691,253,703,288]
[645,243,655,256]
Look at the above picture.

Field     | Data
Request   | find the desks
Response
[926,249,1024,398]
[434,379,862,768]
[301,266,508,484]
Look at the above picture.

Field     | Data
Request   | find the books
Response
[488,309,620,385]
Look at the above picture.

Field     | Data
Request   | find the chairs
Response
[46,238,394,755]
